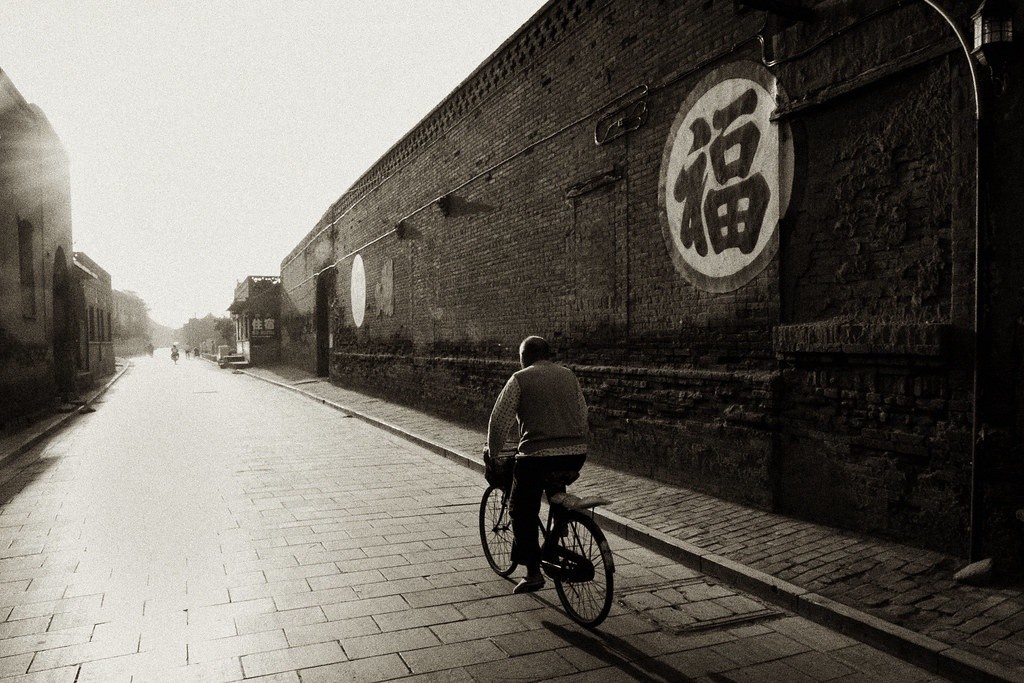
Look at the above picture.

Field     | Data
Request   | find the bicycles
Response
[478,439,616,629]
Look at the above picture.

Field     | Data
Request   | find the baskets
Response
[484,441,520,486]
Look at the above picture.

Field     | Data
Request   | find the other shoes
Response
[560,525,569,537]
[512,576,546,593]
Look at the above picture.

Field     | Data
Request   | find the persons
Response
[488,336,587,593]
[148,341,215,359]
